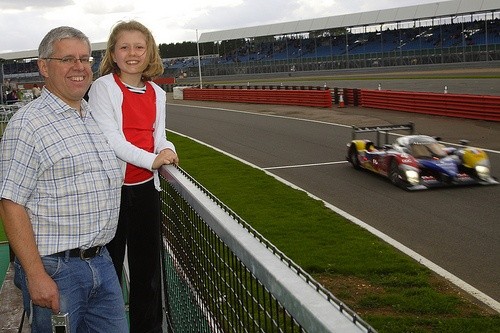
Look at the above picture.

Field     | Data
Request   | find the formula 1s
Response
[345,120,500,192]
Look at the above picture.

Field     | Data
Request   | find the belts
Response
[45,246,106,260]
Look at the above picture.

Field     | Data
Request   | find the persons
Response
[86,20,181,333]
[0,25,131,333]
[7,88,24,105]
[32,83,41,99]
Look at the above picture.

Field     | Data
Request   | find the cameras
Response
[52,312,70,333]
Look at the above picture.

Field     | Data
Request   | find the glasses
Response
[41,55,96,65]
